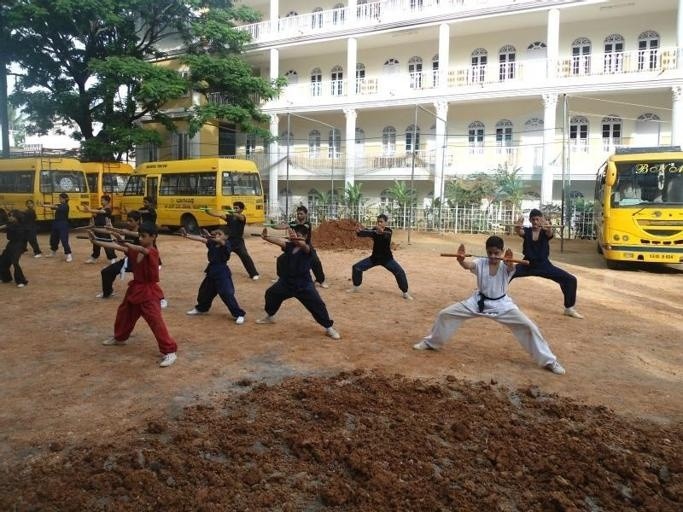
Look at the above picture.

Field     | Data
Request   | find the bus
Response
[590,144,682,266]
[118,160,264,236]
[0,150,93,230]
[76,160,134,223]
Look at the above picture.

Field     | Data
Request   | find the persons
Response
[255,224,341,339]
[272,206,329,288]
[179,226,246,324]
[411,236,566,375]
[511,209,583,319]
[0,195,178,367]
[204,202,260,280]
[346,213,414,301]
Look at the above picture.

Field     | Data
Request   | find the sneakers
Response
[404,292,414,301]
[323,326,341,340]
[247,270,281,284]
[413,340,440,350]
[160,297,168,309]
[186,308,207,316]
[94,292,116,298]
[102,336,129,346]
[545,359,567,375]
[233,311,278,325]
[318,279,359,292]
[160,352,177,368]
[33,250,117,265]
[563,305,584,320]
[17,283,26,288]
